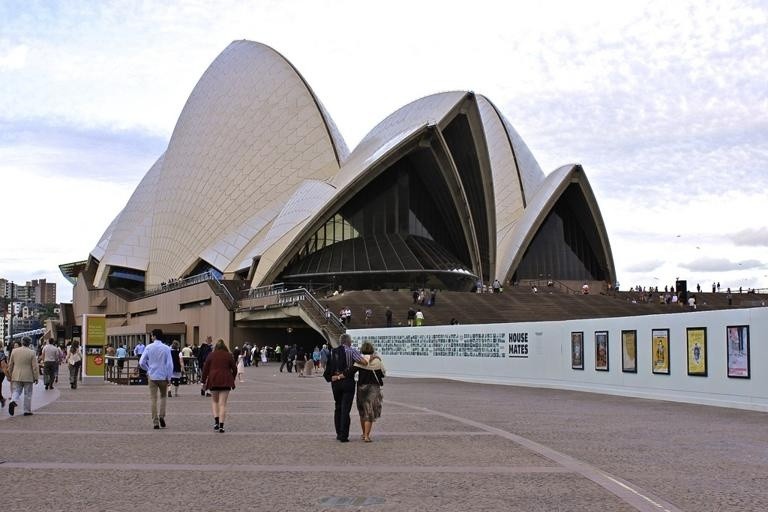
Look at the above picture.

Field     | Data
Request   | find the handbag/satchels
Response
[379,386,384,396]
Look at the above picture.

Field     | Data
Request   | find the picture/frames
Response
[651,328,670,375]
[725,325,751,380]
[621,330,637,373]
[686,326,708,377]
[570,331,584,370]
[594,330,609,372]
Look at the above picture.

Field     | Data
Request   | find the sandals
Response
[361,435,372,442]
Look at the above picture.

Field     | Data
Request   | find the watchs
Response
[337,375,341,381]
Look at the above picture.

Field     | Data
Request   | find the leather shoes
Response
[9,401,17,416]
[24,413,33,416]
[337,437,350,442]
[154,417,166,429]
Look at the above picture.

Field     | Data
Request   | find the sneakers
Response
[214,424,224,432]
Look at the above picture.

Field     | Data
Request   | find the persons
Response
[160,275,188,289]
[200,337,239,434]
[607,280,757,311]
[232,340,329,383]
[547,279,553,286]
[533,284,538,294]
[475,278,502,297]
[385,308,392,327]
[365,306,373,321]
[168,337,212,397]
[0,336,82,417]
[415,308,425,326]
[330,341,385,442]
[323,333,368,443]
[324,304,352,325]
[105,339,127,373]
[138,329,174,431]
[134,339,146,358]
[412,288,436,307]
[581,282,589,294]
[407,307,415,327]
